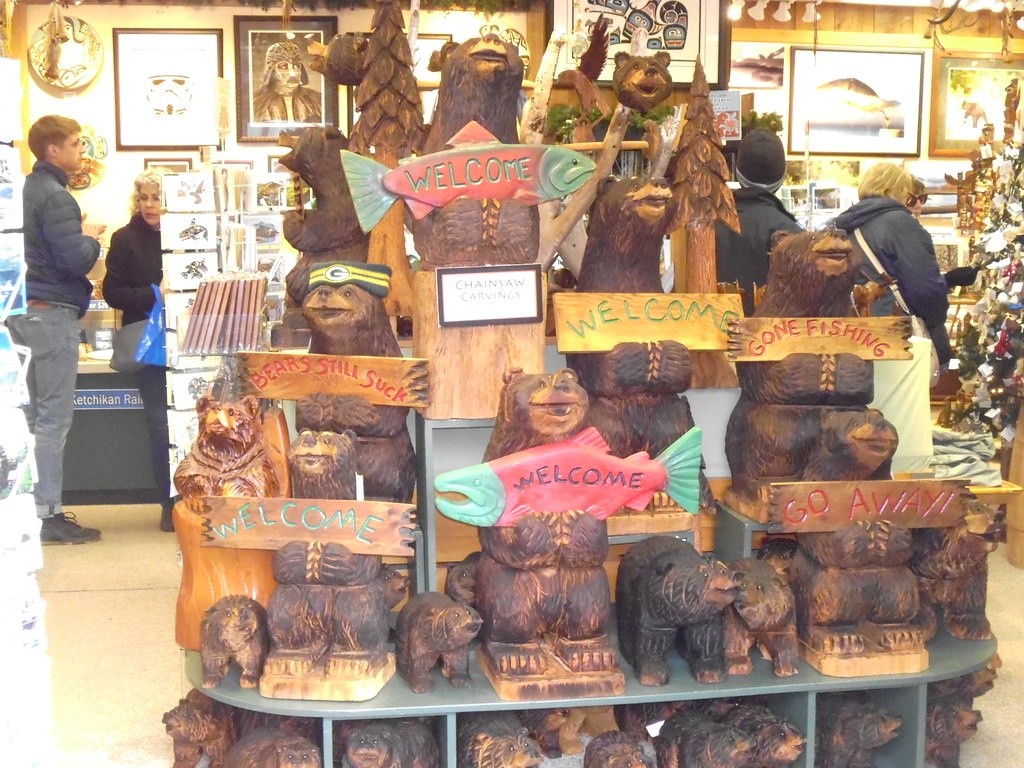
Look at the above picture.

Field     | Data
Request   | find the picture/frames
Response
[212,159,252,172]
[268,155,314,210]
[233,14,338,142]
[144,158,193,174]
[929,50,1024,158]
[544,0,733,92]
[787,46,925,157]
[437,264,543,327]
[405,34,454,90]
[112,27,224,151]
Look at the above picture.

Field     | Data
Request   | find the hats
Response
[735,128,787,192]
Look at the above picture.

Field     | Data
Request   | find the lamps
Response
[747,0,769,20]
[773,0,794,22]
[727,0,745,20]
[802,0,821,22]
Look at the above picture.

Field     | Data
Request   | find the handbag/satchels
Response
[910,315,941,389]
[109,320,162,374]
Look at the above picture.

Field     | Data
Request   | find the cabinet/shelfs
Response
[182,342,997,768]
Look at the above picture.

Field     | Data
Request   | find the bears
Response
[161,31,1004,768]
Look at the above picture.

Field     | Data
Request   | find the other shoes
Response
[39,512,101,545]
[158,504,175,533]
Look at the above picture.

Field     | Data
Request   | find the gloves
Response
[945,268,982,288]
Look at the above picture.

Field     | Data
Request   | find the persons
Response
[717,130,804,318]
[103,168,176,532]
[4,117,107,544]
[838,163,980,365]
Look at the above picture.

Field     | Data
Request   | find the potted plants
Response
[623,107,673,142]
[546,104,581,142]
[592,107,610,141]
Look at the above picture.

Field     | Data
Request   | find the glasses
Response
[905,193,928,207]
